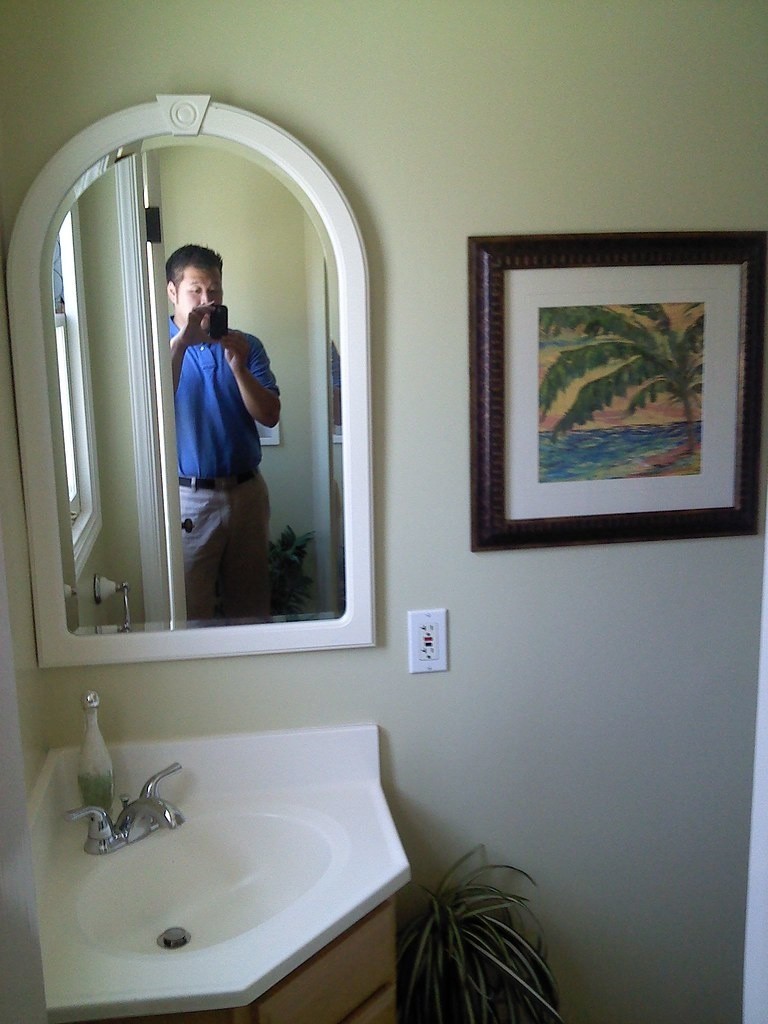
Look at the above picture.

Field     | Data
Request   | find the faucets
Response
[115,794,187,845]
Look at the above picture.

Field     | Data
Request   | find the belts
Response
[179,469,258,489]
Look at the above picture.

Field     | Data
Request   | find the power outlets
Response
[406,608,448,676]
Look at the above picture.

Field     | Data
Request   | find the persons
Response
[166,245,281,620]
[332,343,341,581]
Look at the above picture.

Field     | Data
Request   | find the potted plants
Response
[398,841,568,1024]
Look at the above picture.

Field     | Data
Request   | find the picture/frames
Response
[468,229,758,553]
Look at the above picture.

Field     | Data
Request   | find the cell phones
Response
[209,305,228,340]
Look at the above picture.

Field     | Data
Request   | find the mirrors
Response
[4,92,372,668]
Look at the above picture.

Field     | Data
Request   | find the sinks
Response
[76,806,349,956]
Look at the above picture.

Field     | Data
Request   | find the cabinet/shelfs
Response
[237,893,398,1024]
